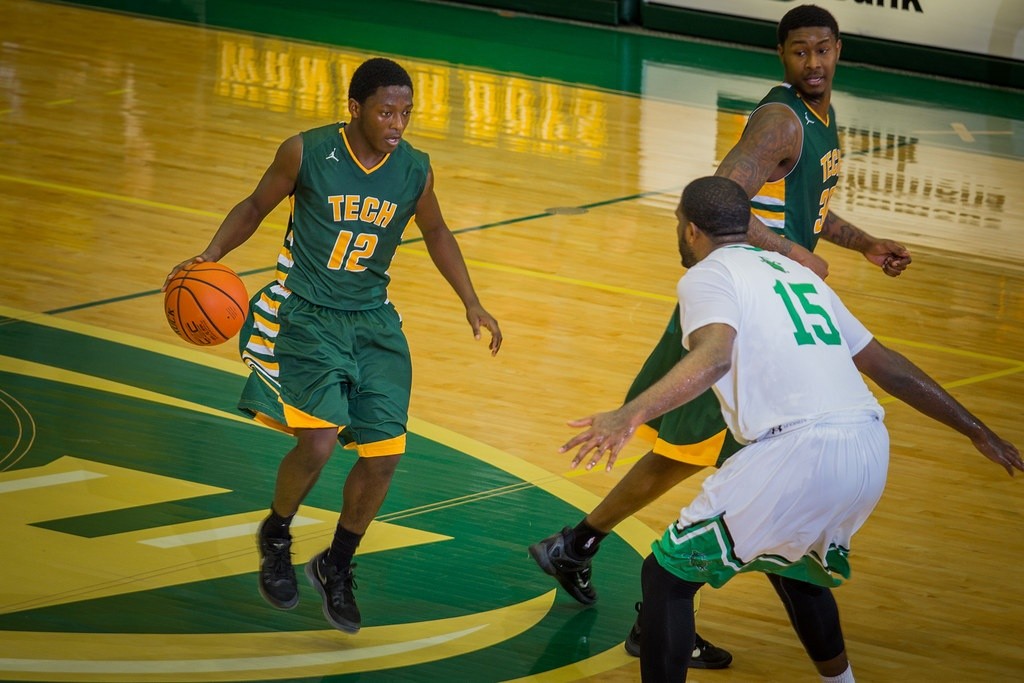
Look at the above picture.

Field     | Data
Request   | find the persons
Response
[559,175,1024,683]
[527,4,911,671]
[160,58,503,635]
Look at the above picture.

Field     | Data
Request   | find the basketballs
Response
[164,261,250,347]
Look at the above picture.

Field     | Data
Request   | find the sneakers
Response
[529,525,599,605]
[305,549,364,635]
[626,601,732,669]
[256,515,299,611]
